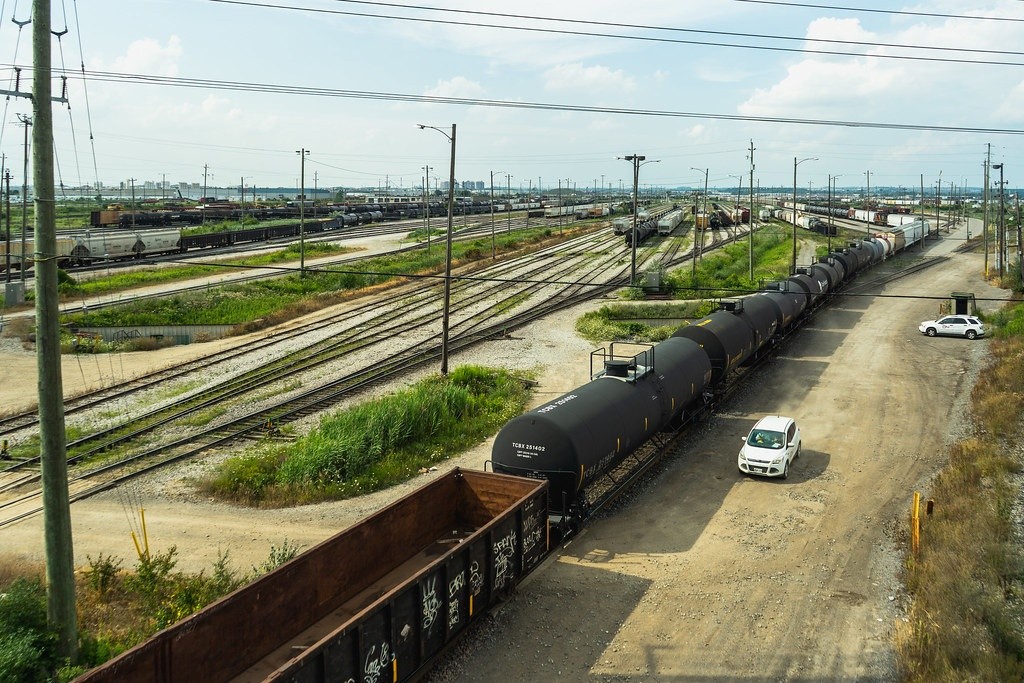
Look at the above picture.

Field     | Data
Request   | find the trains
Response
[91,194,594,214]
[0,211,384,271]
[862,205,911,214]
[696,201,750,230]
[754,196,888,237]
[70,219,932,680]
[614,202,687,246]
[544,201,650,219]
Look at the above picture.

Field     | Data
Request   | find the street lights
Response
[992,164,1003,280]
[828,174,846,255]
[749,179,759,234]
[593,180,600,204]
[727,175,741,244]
[944,181,953,235]
[746,156,753,282]
[613,157,662,280]
[792,158,819,273]
[831,178,839,224]
[624,156,645,286]
[689,168,708,262]
[1010,194,1024,287]
[413,123,455,373]
[964,178,967,221]
[863,173,873,237]
[295,150,310,279]
[421,167,433,256]
[491,171,505,259]
[935,187,937,217]
[201,173,210,226]
[505,176,513,236]
[808,182,814,217]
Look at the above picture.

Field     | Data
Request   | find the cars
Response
[107,202,125,211]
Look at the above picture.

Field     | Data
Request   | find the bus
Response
[287,201,315,209]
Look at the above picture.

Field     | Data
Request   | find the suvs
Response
[919,314,988,339]
[737,416,802,479]
